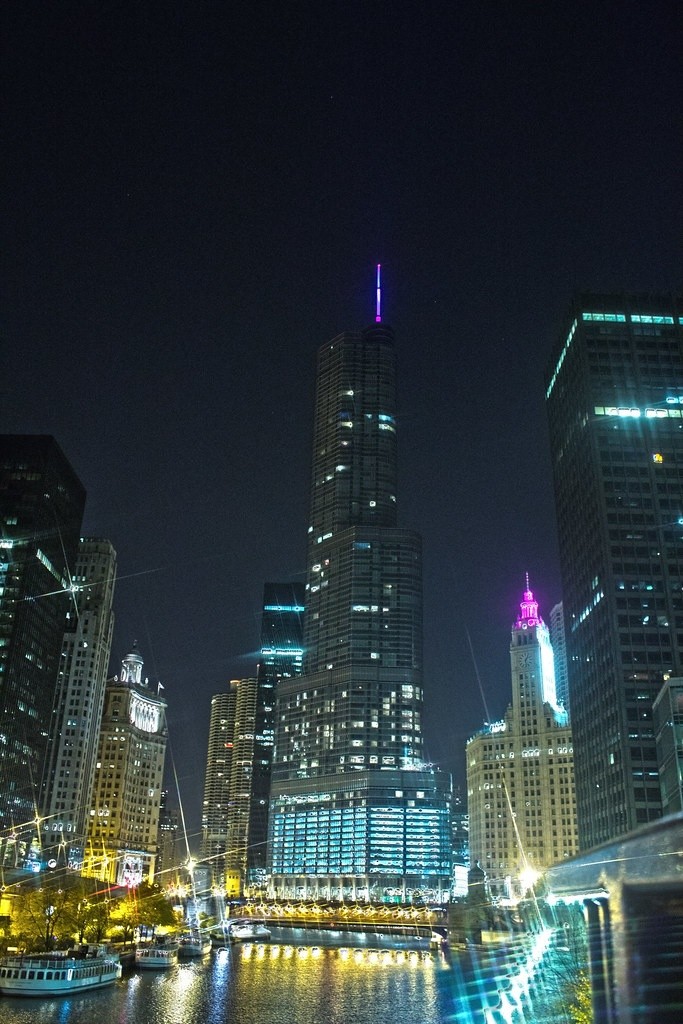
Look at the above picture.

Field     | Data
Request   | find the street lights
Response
[46,858,56,952]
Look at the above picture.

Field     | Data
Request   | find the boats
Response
[136,938,180,969]
[0,945,121,998]
[230,922,271,941]
[171,926,211,955]
